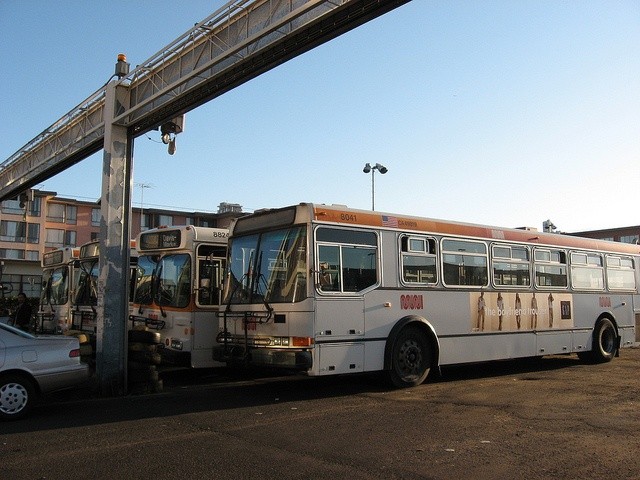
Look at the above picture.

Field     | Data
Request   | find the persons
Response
[547,293,554,328]
[530,293,538,329]
[11,292,34,332]
[514,293,522,329]
[497,293,504,331]
[476,292,486,332]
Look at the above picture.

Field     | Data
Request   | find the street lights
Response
[136,183,150,227]
[363,162,387,213]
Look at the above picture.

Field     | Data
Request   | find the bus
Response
[137,224,228,369]
[72,238,137,331]
[36,247,80,331]
[212,201,640,386]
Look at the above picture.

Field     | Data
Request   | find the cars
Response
[0,323,82,421]
